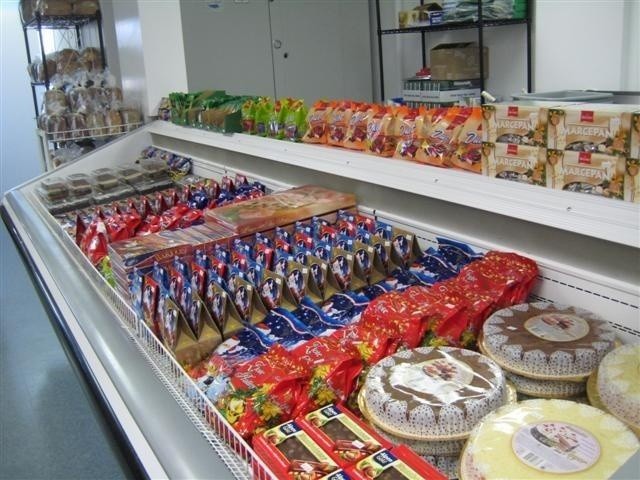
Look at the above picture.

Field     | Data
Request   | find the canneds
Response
[20,1,140,141]
